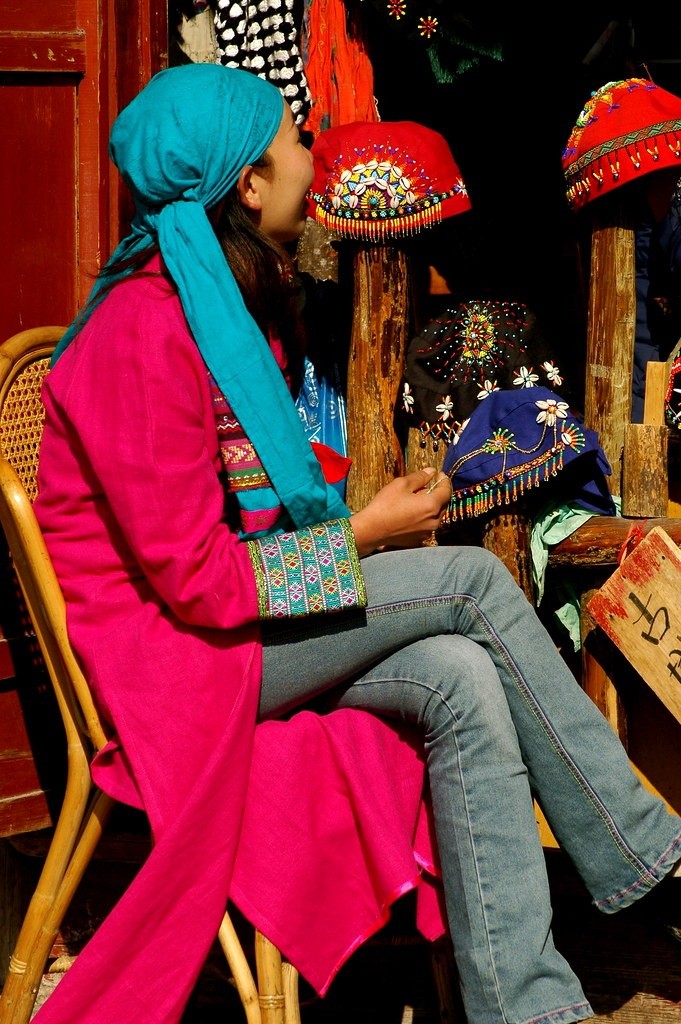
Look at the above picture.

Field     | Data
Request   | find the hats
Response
[562,78,681,210]
[441,391,616,528]
[304,121,472,244]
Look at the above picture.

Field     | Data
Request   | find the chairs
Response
[0,324,461,1023]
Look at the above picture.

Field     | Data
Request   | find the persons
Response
[34,63,681,1024]
[631,174,681,423]
